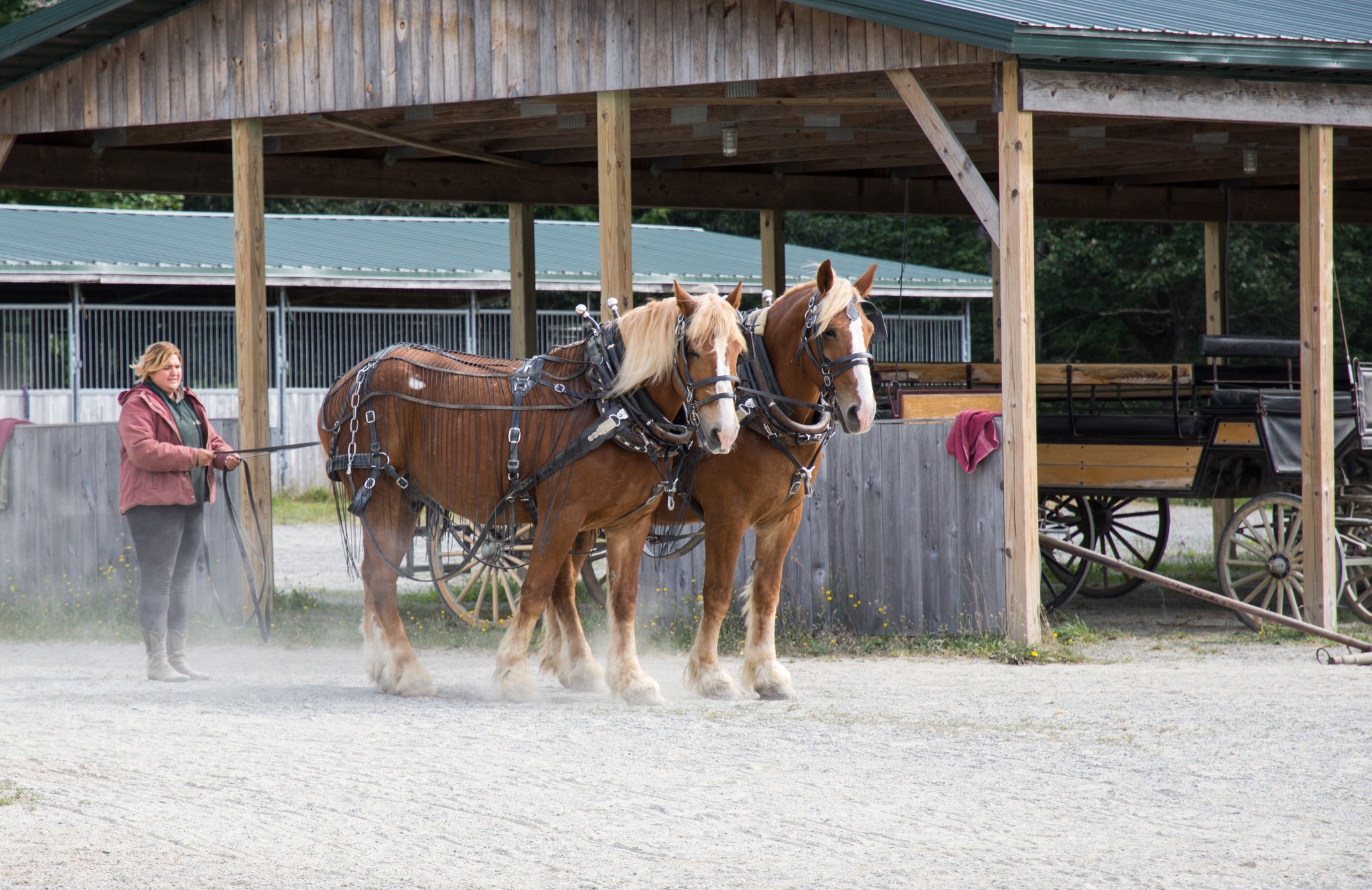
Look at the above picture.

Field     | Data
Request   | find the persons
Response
[118,342,241,683]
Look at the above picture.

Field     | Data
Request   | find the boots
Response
[167,628,209,681]
[144,626,188,682]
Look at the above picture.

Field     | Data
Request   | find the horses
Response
[319,280,747,704]
[535,259,879,701]
